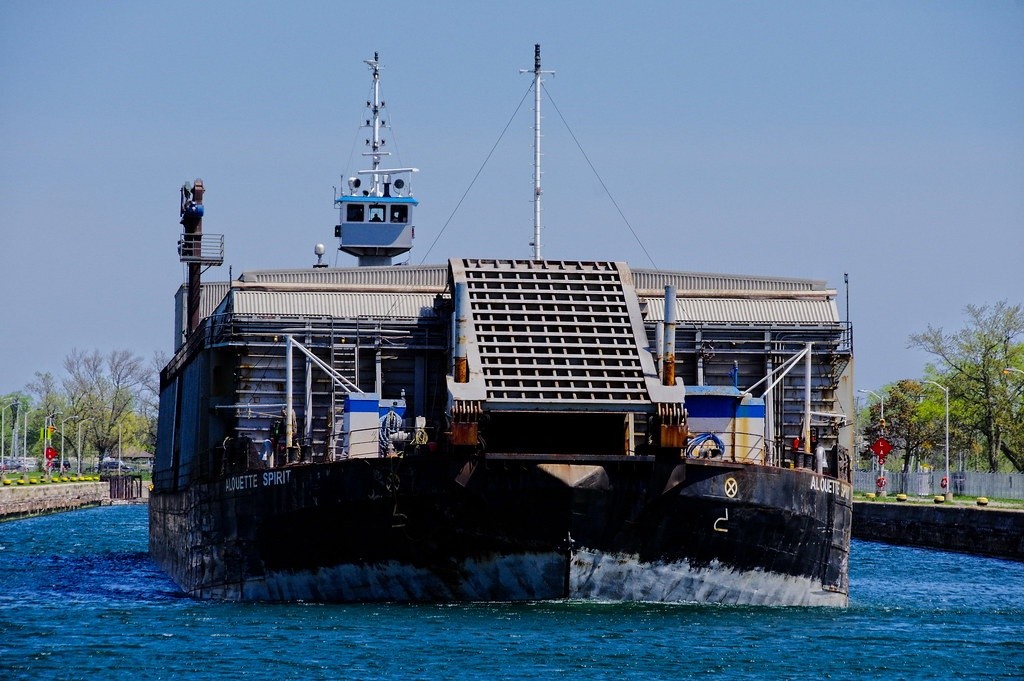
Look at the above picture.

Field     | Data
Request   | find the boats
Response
[147,43,858,608]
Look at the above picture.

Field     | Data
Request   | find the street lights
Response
[919,381,953,502]
[43,412,64,471]
[23,407,42,469]
[78,418,94,473]
[2,403,21,472]
[858,389,886,497]
[60,416,79,472]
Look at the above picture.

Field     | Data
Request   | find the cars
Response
[0,459,27,473]
[101,461,132,472]
[48,460,72,472]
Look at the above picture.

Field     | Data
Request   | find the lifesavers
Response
[876,477,887,487]
[940,477,947,488]
